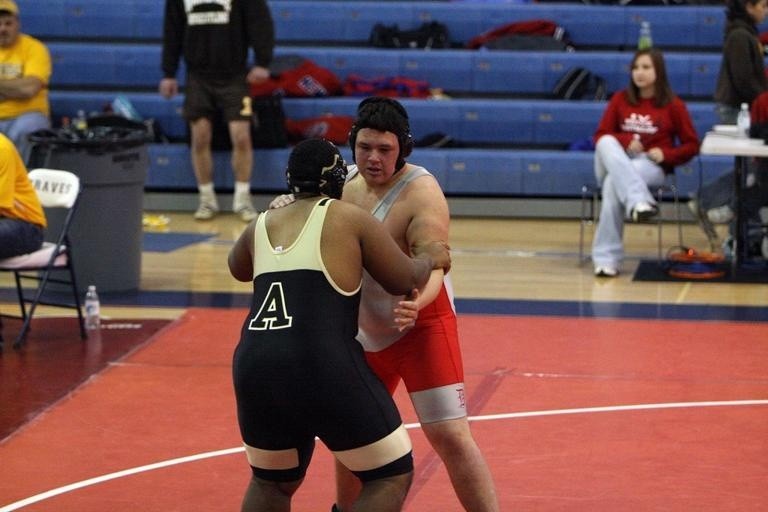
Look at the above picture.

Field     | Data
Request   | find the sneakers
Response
[595,267,617,277]
[232,203,258,222]
[194,201,219,219]
[632,203,659,224]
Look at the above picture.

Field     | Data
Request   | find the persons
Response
[269,97,498,511]
[592,48,699,278]
[0,133,47,349]
[0,0,53,166]
[159,1,275,223]
[686,0,766,225]
[228,138,452,512]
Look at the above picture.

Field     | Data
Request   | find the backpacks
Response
[549,69,609,102]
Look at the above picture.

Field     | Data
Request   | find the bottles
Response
[737,102,751,140]
[86,285,101,331]
[639,20,652,50]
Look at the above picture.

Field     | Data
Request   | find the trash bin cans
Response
[27,121,147,294]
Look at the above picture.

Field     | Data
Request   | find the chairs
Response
[580,122,693,268]
[1,168,86,349]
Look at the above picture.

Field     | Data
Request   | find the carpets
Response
[630,258,767,283]
[139,230,216,253]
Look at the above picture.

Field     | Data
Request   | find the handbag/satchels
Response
[365,21,447,49]
[184,93,287,150]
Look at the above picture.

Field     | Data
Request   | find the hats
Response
[0,0,18,16]
[286,138,348,199]
[350,96,413,169]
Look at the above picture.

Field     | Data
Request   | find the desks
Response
[697,122,767,281]
